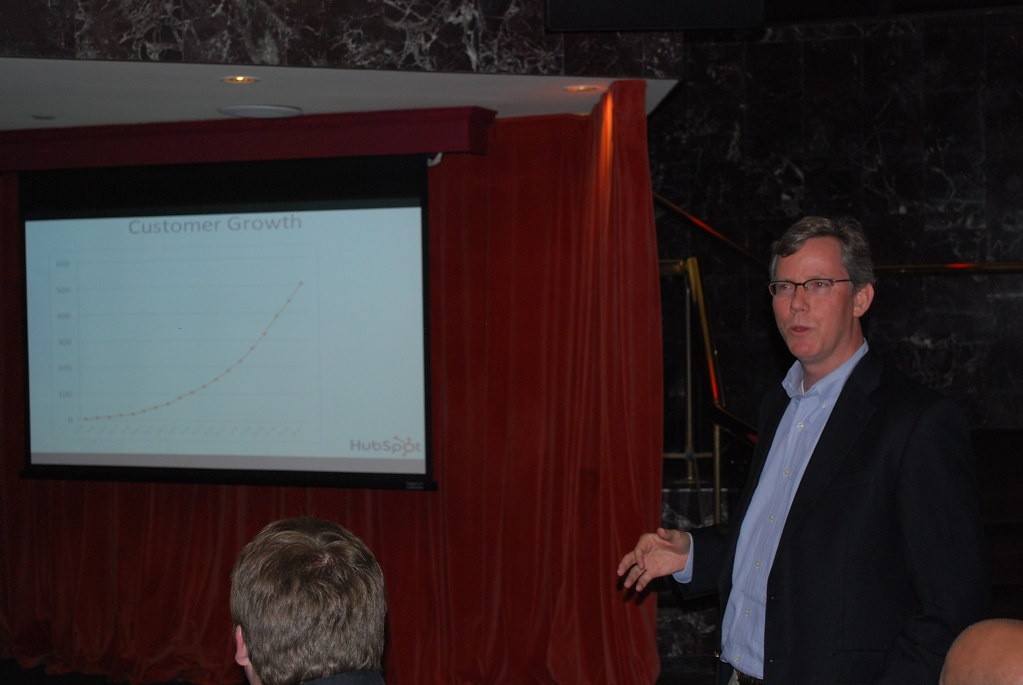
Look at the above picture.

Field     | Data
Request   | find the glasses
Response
[769,278,857,297]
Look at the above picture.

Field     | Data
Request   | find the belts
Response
[715,653,763,685]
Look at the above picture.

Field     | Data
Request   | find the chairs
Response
[686,255,761,526]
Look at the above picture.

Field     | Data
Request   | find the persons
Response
[940,619,1023,685]
[617,218,993,685]
[231,519,387,685]
